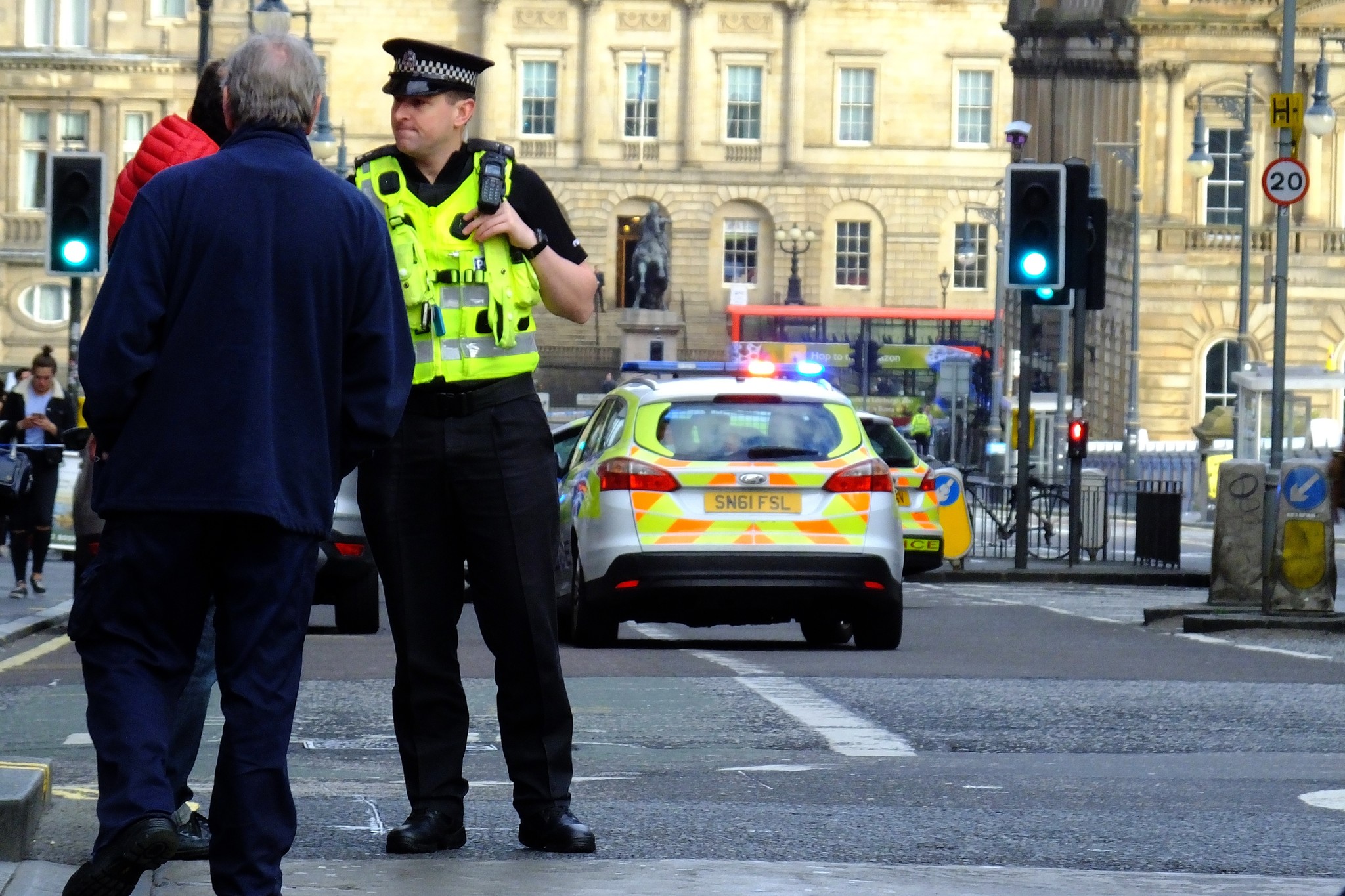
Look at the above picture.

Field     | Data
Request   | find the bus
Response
[720,303,1004,431]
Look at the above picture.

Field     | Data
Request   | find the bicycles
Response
[940,461,1084,563]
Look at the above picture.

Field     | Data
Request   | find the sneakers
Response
[9,581,28,598]
[173,810,212,860]
[519,807,597,855]
[0,544,11,558]
[55,819,180,895]
[30,575,47,594]
[384,806,467,854]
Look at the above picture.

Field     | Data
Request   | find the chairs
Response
[795,331,938,344]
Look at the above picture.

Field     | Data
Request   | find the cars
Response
[556,359,902,650]
[854,410,946,577]
[310,463,380,635]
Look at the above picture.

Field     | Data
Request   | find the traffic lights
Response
[1068,421,1088,459]
[1027,287,1075,311]
[1005,162,1065,291]
[44,150,109,280]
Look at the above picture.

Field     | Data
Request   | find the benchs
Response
[657,412,823,462]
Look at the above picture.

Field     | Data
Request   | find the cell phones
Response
[31,413,43,421]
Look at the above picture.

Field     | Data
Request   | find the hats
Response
[379,38,496,96]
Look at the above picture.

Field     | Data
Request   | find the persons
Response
[61,35,418,896]
[1,343,75,600]
[77,53,266,863]
[317,36,603,853]
[12,363,36,394]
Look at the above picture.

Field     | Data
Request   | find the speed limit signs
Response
[1261,156,1309,206]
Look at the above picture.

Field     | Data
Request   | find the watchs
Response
[522,228,550,259]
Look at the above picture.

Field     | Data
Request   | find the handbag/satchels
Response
[0,420,32,493]
